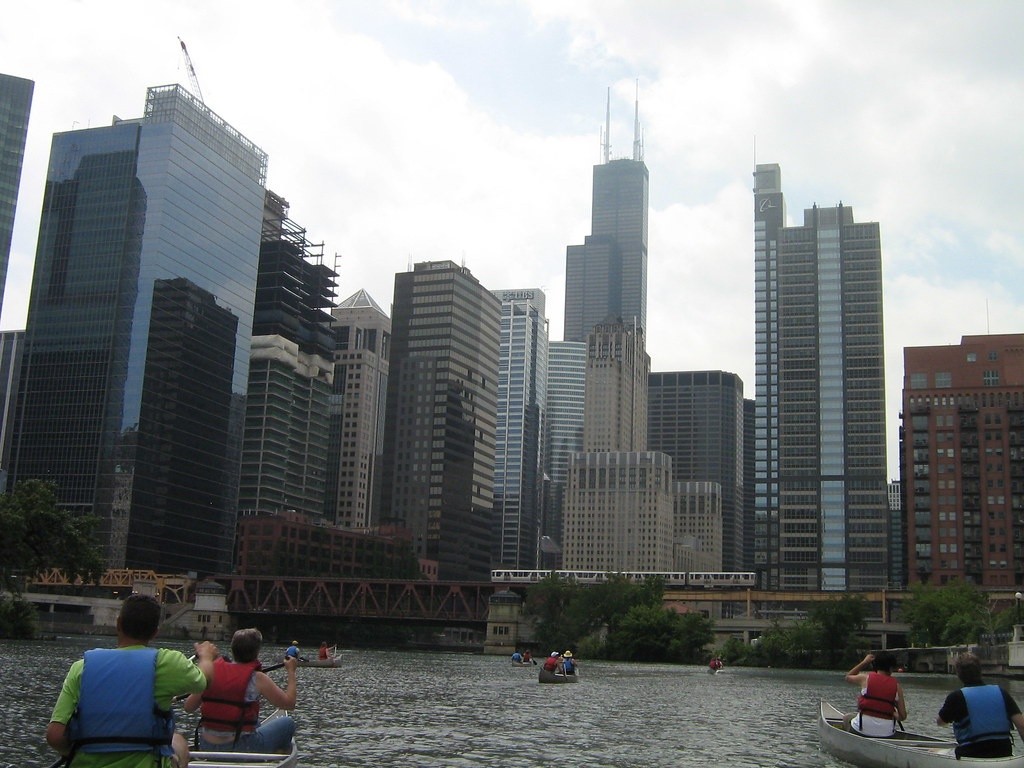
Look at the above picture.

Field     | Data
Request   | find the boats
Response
[817,695,1024,768]
[304,654,344,668]
[46,708,298,767]
[512,658,534,667]
[538,664,580,683]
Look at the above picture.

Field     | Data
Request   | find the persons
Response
[845,650,907,738]
[522,649,532,662]
[543,651,564,675]
[319,641,337,661]
[47,593,217,768]
[285,640,303,662]
[562,650,578,674]
[936,654,1024,759]
[183,629,298,762]
[511,649,522,663]
[709,657,723,670]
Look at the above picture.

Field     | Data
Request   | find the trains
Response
[490,569,758,591]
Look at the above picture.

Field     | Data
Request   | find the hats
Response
[564,650,573,658]
[551,651,559,657]
[292,640,298,645]
[955,653,984,676]
